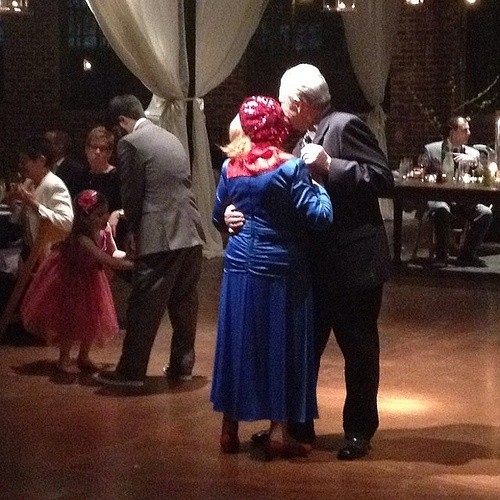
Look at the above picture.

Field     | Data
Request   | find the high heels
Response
[264,438,315,462]
[220,431,240,453]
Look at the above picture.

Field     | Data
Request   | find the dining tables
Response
[372,172,500,268]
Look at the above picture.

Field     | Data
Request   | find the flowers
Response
[77,190,98,209]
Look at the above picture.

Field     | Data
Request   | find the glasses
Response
[88,142,111,152]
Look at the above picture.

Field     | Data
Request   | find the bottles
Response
[442,138,454,182]
[397,158,414,183]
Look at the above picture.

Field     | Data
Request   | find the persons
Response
[19,189,134,375]
[73,126,122,210]
[38,131,75,189]
[210,95,332,460]
[93,95,201,387]
[8,137,73,251]
[225,63,395,460]
[407,115,487,267]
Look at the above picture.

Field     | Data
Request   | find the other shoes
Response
[55,360,81,374]
[456,257,485,267]
[92,370,145,387]
[163,366,192,381]
[251,423,315,445]
[78,358,100,373]
[431,255,448,267]
[338,443,368,460]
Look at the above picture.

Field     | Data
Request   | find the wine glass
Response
[468,155,479,185]
[418,154,430,182]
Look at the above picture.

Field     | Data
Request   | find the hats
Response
[473,144,495,162]
[239,95,290,148]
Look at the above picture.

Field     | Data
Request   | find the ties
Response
[304,136,311,144]
[453,148,459,177]
